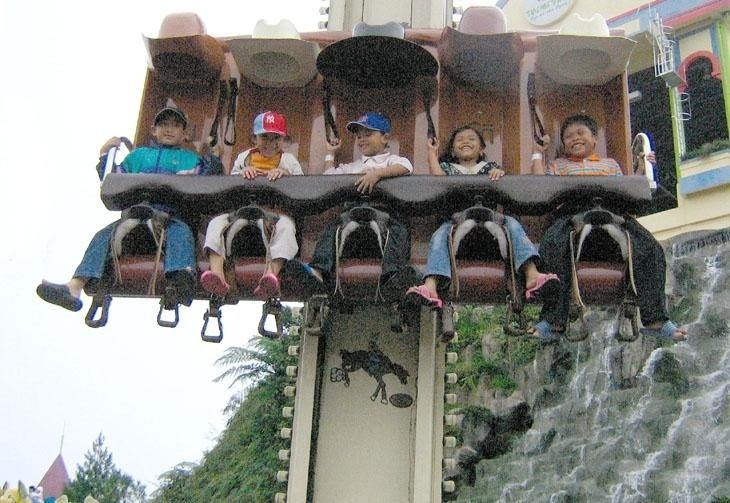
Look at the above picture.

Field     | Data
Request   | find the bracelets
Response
[531,153,543,160]
[325,155,334,162]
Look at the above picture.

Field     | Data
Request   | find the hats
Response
[253,110,288,138]
[153,107,186,127]
[344,112,392,136]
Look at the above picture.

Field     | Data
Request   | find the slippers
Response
[166,266,196,308]
[379,266,415,301]
[405,284,443,311]
[37,280,83,313]
[525,268,561,301]
[201,270,232,297]
[639,321,689,342]
[253,274,278,299]
[525,320,563,343]
[288,260,326,289]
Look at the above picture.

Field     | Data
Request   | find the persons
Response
[407,126,561,309]
[284,112,417,294]
[200,110,304,301]
[37,106,225,312]
[529,114,688,341]
